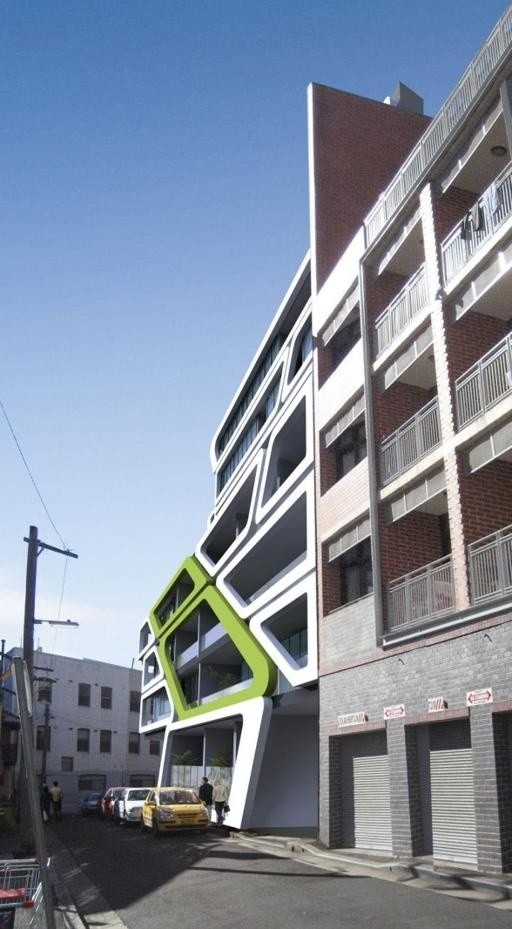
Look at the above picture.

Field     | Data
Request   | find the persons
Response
[40,781,64,823]
[199,777,227,825]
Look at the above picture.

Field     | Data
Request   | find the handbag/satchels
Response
[224,805,230,812]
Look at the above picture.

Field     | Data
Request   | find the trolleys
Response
[0,857,50,929]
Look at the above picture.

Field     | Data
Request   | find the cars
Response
[80,787,209,838]
